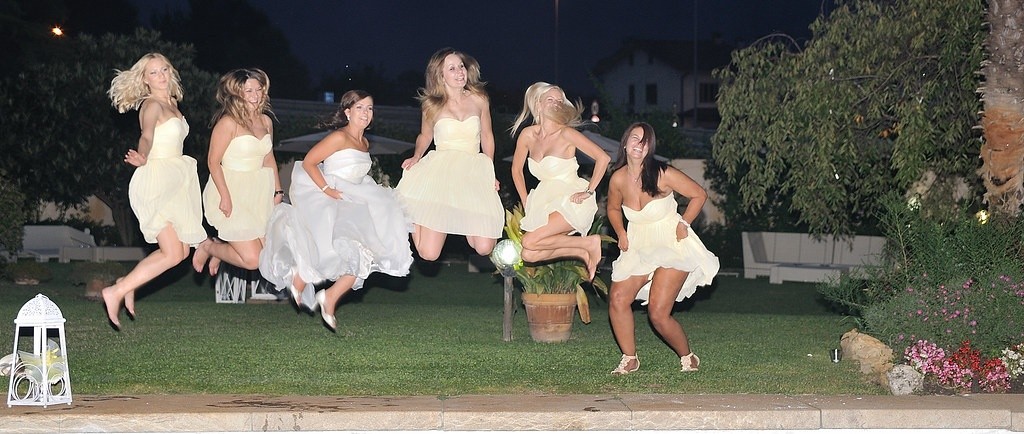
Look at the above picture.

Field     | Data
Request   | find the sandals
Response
[681,352,700,371]
[611,353,640,376]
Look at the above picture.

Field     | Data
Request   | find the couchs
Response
[741,230,899,285]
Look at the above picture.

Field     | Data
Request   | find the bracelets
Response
[678,218,691,228]
[322,183,329,191]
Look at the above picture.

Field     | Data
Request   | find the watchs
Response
[274,188,285,197]
[584,187,594,195]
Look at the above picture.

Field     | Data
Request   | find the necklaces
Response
[624,161,645,184]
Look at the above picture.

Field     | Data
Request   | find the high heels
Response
[311,290,336,329]
[290,268,302,306]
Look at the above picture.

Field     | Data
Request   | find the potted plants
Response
[503,200,618,345]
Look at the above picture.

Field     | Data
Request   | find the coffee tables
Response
[770,262,849,288]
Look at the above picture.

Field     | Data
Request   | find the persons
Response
[100,51,208,326]
[508,81,611,281]
[394,48,506,261]
[606,122,720,377]
[258,87,414,331]
[191,67,286,275]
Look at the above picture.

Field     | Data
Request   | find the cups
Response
[829,349,842,364]
